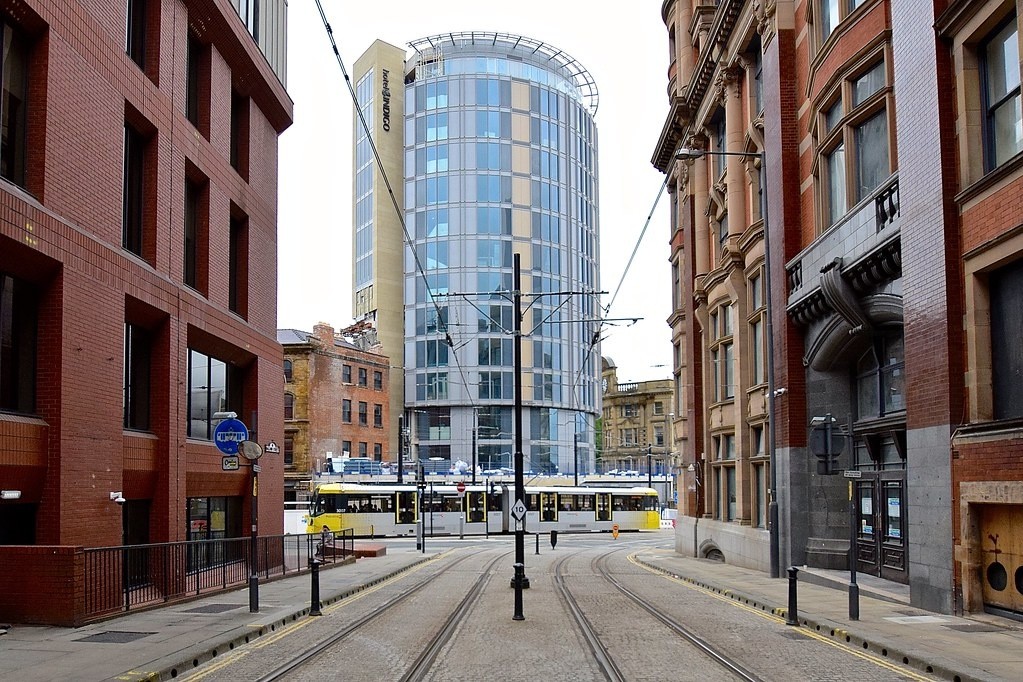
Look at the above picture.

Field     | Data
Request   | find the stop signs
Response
[457,482,465,492]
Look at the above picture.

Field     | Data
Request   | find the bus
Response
[307,483,660,539]
[284,501,324,536]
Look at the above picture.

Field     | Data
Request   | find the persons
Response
[315,525,333,555]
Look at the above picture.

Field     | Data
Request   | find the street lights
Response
[665,413,674,509]
[674,148,780,579]
